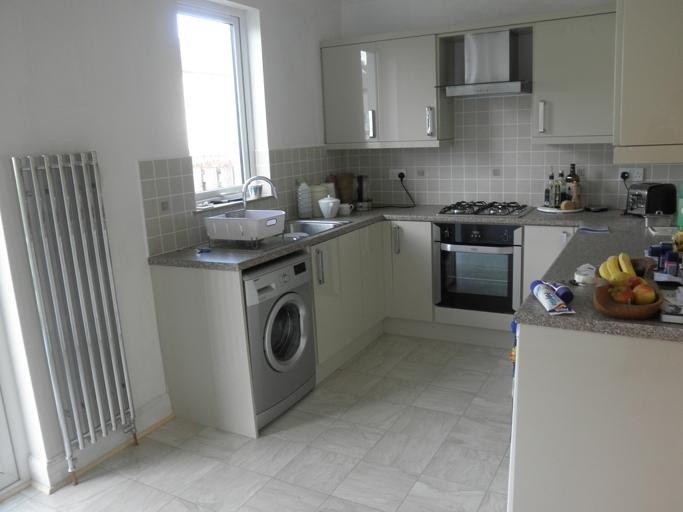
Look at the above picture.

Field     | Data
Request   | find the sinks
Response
[282,218,339,240]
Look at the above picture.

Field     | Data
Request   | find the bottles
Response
[319,173,352,204]
[647,240,682,277]
[295,181,311,219]
[544,164,581,208]
[310,185,327,218]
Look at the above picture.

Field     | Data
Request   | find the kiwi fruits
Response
[561,200,575,209]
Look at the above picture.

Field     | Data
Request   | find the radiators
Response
[10,149,139,487]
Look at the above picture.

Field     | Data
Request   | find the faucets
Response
[241,175,280,209]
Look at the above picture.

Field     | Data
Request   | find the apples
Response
[610,274,657,303]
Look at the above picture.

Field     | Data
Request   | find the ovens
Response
[433,241,520,317]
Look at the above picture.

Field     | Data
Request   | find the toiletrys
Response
[528,278,577,315]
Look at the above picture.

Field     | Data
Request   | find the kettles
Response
[355,175,372,212]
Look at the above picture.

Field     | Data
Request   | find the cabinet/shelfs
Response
[610,0,683,166]
[522,225,575,300]
[318,42,379,151]
[528,9,618,146]
[380,33,454,151]
[305,221,361,378]
[361,219,432,338]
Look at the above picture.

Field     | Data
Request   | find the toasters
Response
[626,182,676,217]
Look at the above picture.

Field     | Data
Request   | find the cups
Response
[340,203,354,215]
[249,184,263,198]
[318,195,340,218]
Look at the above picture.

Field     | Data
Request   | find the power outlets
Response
[617,167,643,182]
[387,168,406,181]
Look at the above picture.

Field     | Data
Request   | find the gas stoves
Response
[439,200,533,217]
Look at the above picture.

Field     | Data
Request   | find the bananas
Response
[600,252,636,279]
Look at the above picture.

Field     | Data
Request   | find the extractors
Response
[445,30,532,101]
